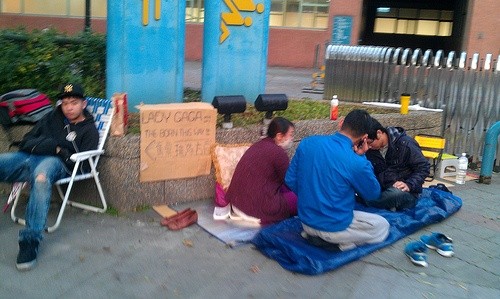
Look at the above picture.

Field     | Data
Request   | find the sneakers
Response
[16,239,39,270]
[420,232,454,257]
[404,241,428,267]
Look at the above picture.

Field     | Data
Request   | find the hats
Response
[58,84,83,99]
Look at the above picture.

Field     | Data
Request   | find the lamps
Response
[212,95,246,128]
[253,93,289,125]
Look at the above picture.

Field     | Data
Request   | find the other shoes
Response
[168,210,198,230]
[161,208,192,226]
[430,184,453,194]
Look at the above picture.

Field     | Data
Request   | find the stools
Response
[436,154,460,179]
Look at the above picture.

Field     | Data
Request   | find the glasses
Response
[367,138,376,146]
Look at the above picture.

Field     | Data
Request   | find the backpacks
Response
[0,89,54,129]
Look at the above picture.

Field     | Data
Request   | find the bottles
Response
[456,153,468,185]
[330,95,338,120]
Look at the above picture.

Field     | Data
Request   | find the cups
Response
[400,93,411,115]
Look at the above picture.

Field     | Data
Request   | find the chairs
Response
[10,97,115,233]
[414,134,446,181]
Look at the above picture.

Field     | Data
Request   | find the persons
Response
[285,109,391,251]
[0,83,99,270]
[355,116,431,211]
[224,117,298,227]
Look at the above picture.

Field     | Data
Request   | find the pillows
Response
[210,142,253,192]
